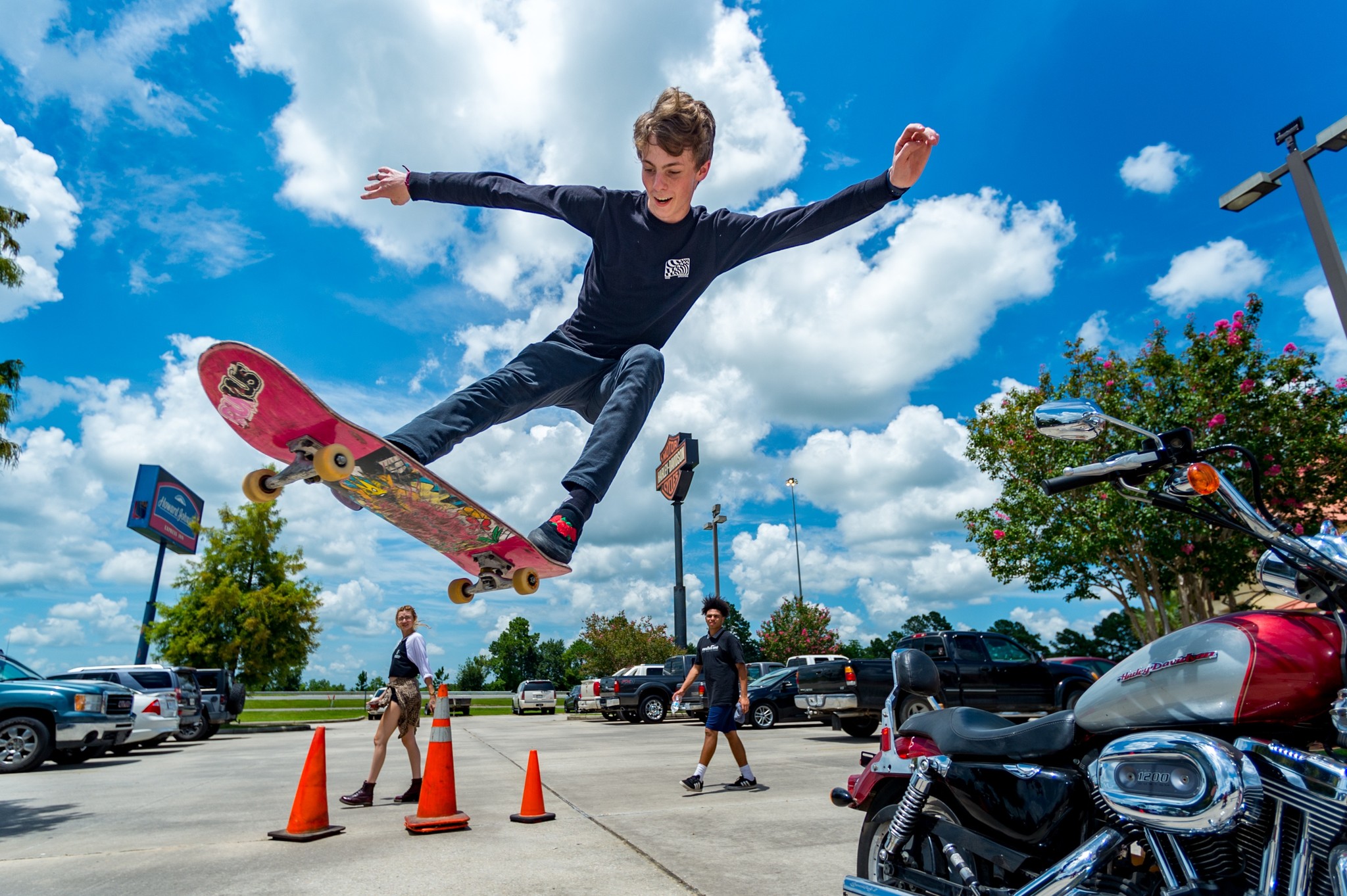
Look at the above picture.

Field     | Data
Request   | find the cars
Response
[739,666,832,729]
[366,687,389,720]
[1043,656,1118,679]
[107,688,181,755]
[564,663,665,714]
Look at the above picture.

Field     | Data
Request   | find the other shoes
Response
[331,488,364,511]
[528,500,587,564]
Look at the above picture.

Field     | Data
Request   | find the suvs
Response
[172,667,246,741]
[0,648,137,774]
[45,665,204,746]
[511,679,558,715]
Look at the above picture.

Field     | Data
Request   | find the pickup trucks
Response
[599,654,704,724]
[676,662,785,724]
[793,630,1101,739]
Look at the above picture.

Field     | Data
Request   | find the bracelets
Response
[429,691,435,696]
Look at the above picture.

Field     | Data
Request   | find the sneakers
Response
[680,774,704,792]
[726,774,757,790]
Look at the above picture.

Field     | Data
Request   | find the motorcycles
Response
[828,391,1347,895]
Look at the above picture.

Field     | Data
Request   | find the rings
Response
[375,173,380,181]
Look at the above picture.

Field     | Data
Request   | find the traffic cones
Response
[267,725,346,841]
[509,749,556,823]
[404,683,473,833]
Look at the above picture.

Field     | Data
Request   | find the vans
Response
[786,655,849,668]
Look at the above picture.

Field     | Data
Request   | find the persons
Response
[360,84,938,564]
[339,606,436,807]
[672,593,757,792]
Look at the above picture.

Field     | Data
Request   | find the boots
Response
[394,777,422,802]
[340,780,376,807]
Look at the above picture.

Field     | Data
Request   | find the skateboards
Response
[197,340,573,605]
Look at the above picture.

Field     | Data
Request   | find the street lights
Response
[703,504,728,599]
[785,477,803,634]
[1217,115,1347,338]
